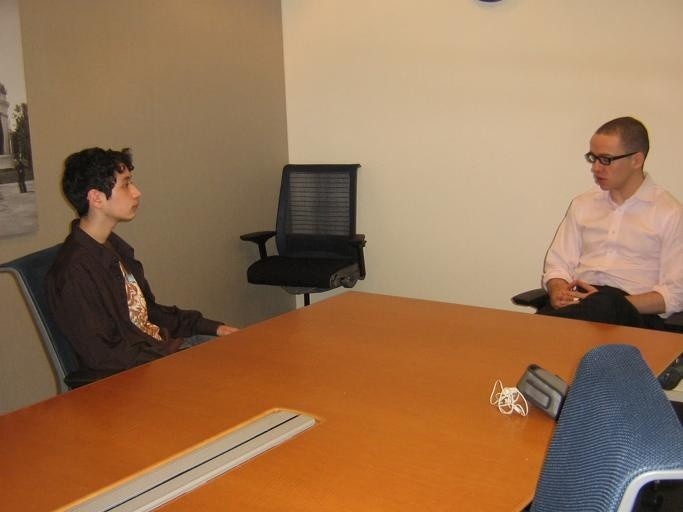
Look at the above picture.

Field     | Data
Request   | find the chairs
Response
[1,244,129,396]
[525,342,682,512]
[238,162,366,308]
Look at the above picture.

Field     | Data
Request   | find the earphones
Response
[489,379,529,417]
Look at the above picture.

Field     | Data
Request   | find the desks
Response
[0,289,682,512]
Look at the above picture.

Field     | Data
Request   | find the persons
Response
[16,161,27,193]
[534,117,683,330]
[48,147,240,390]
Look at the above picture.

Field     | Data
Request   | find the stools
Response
[514,287,682,390]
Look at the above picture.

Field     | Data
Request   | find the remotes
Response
[658,353,683,390]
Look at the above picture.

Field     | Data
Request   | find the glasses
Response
[584,152,637,165]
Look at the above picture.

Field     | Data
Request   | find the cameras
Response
[515,364,569,422]
